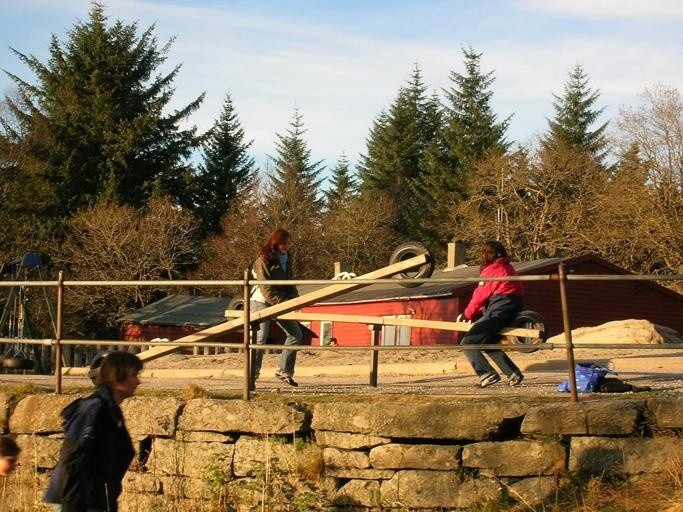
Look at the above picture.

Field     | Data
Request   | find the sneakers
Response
[274,368,299,387]
[475,370,502,389]
[507,373,525,387]
[248,375,257,391]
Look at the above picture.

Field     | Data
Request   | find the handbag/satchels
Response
[594,377,652,394]
[555,361,619,394]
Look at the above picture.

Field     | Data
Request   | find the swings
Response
[0,263,36,369]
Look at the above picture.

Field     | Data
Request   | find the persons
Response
[455,241,525,388]
[0,436,21,477]
[246,229,302,394]
[40,351,153,512]
